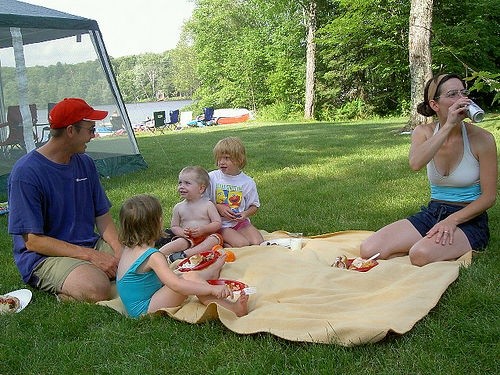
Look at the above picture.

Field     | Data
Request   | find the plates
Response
[5,289,33,313]
[332,258,378,271]
[206,280,247,292]
[260,237,296,249]
[176,251,221,270]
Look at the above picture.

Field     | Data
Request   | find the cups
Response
[290,233,303,250]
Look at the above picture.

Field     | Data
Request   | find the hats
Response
[48,97,108,128]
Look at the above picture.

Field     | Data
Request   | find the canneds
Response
[461,99,485,122]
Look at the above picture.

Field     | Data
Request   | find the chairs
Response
[144,111,166,135]
[33,103,57,147]
[0,104,38,158]
[109,116,125,133]
[166,109,181,131]
[198,107,217,124]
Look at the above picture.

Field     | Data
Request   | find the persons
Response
[8,97,124,305]
[116,195,250,319]
[360,73,497,266]
[157,166,224,264]
[201,137,264,248]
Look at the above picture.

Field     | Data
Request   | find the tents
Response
[0,0,148,215]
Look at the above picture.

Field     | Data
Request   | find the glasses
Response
[434,89,469,99]
[73,125,95,135]
